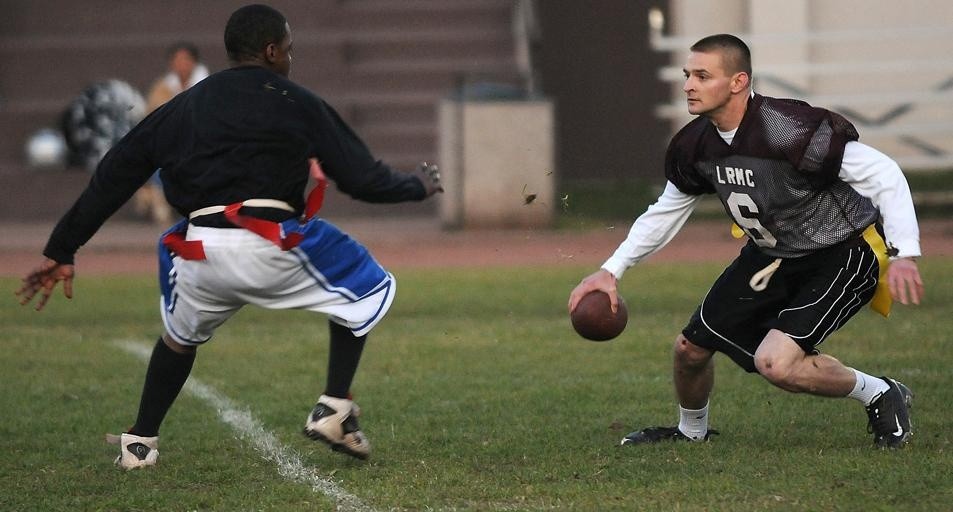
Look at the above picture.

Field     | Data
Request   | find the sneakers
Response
[105,431,160,469]
[865,376,915,450]
[619,425,720,447]
[303,393,370,459]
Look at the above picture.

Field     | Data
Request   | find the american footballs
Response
[571,290,628,341]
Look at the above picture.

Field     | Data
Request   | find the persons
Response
[125,42,211,223]
[11,4,444,473]
[567,32,925,450]
